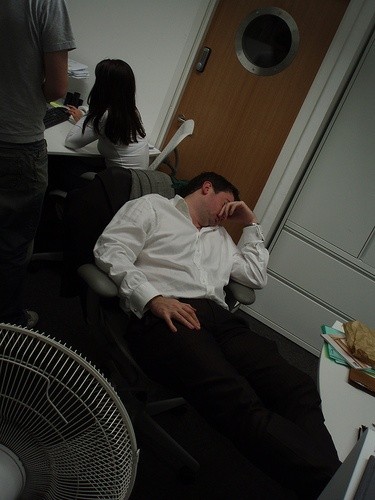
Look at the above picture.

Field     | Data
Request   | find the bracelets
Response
[242,223,261,227]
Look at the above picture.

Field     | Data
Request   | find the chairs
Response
[38,168,228,480]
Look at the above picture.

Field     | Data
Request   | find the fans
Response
[0,323,140,500]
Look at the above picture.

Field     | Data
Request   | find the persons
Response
[0,0,77,329]
[64,59,149,169]
[93,172,324,500]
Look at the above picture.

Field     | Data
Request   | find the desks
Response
[318,320,375,463]
